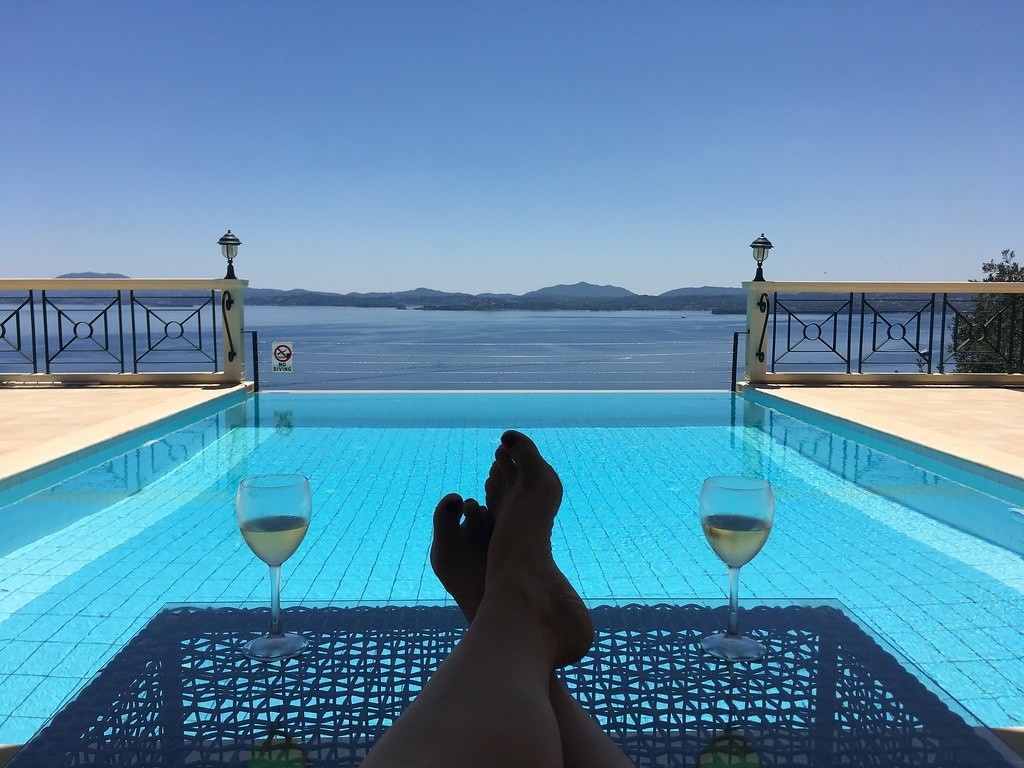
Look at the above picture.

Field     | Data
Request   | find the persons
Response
[356,430,636,768]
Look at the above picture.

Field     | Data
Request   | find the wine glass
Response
[700,476,774,663]
[236,473,312,664]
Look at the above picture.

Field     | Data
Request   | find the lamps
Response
[750,234,774,281]
[217,230,243,279]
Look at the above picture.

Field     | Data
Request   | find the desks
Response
[3,597,1024,768]
